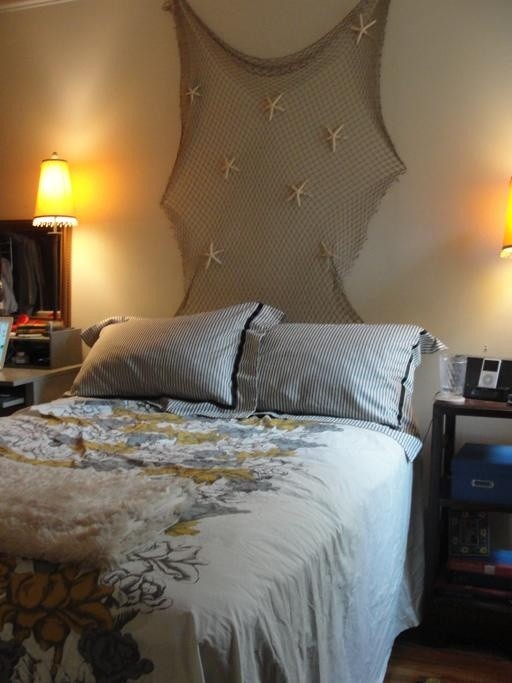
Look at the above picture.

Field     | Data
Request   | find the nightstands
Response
[0,326,79,418]
[426,388,511,661]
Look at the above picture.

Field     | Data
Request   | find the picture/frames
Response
[0,218,70,328]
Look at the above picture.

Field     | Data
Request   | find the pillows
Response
[257,318,445,462]
[63,302,284,422]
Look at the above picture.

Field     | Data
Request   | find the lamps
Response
[33,151,75,235]
[499,177,512,258]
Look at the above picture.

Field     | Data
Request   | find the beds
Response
[0,395,426,683]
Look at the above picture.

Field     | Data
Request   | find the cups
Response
[438,352,467,401]
